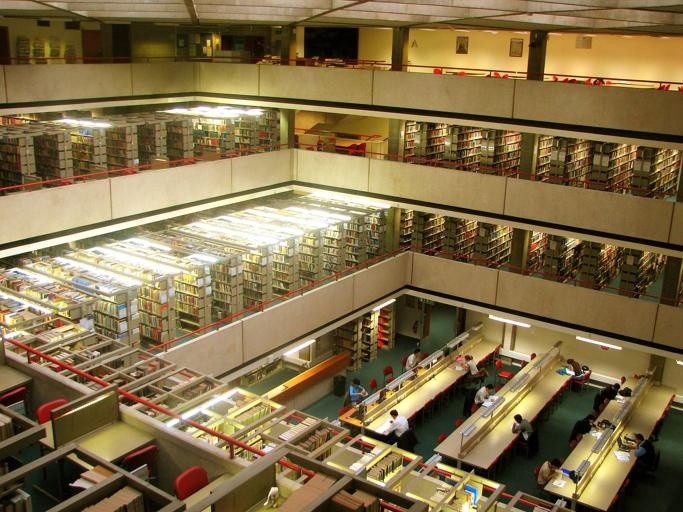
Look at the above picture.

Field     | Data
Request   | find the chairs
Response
[172,465,210,500]
[0,386,28,434]
[291,125,386,162]
[119,443,158,482]
[36,398,72,479]
[431,64,681,94]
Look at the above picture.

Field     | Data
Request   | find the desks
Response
[0,340,33,396]
[180,472,236,512]
[254,51,389,71]
[53,391,155,464]
[210,464,285,512]
[36,382,119,451]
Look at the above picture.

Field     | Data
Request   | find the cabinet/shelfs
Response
[15,34,68,64]
[388,116,682,203]
[0,89,280,191]
[396,202,682,311]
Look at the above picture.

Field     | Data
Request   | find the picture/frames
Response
[508,36,523,57]
[455,35,469,54]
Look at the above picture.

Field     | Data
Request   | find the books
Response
[0,37,683,511]
